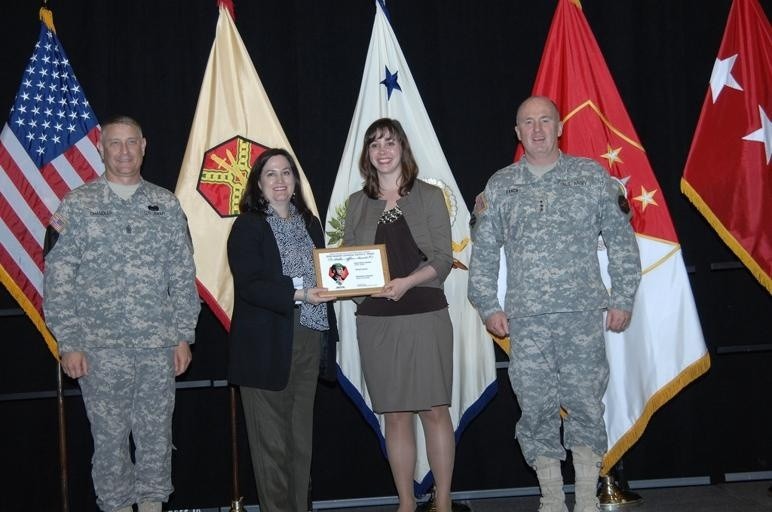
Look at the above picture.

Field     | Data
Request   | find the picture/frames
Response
[311,242,391,301]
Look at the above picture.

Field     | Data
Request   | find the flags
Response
[482,0,712,477]
[679,0,772,291]
[324,0,498,499]
[173,0,324,331]
[0,8,105,361]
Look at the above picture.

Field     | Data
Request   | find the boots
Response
[571,448,602,512]
[119,501,163,512]
[534,456,567,512]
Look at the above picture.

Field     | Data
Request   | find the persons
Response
[341,118,457,512]
[228,150,338,512]
[42,117,202,512]
[468,96,641,512]
